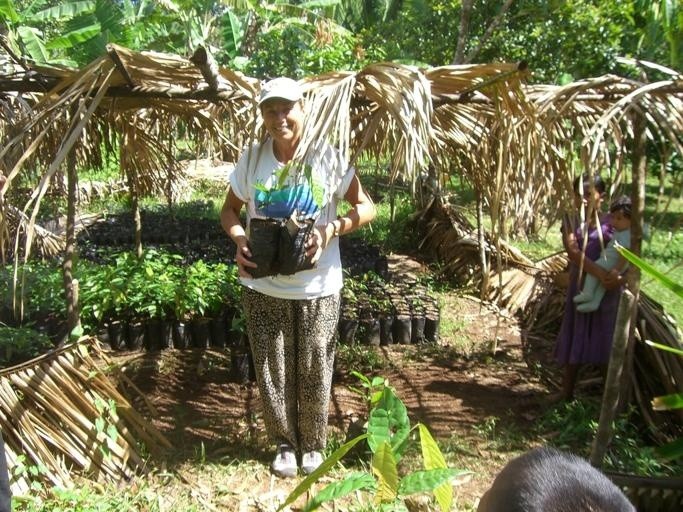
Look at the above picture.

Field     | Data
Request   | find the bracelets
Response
[329,221,339,239]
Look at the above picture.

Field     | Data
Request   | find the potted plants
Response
[0,247,443,350]
[343,370,395,458]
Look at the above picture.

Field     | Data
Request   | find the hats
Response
[255,74,302,108]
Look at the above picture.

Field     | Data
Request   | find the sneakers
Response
[269,440,327,480]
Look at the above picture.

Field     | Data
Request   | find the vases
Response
[249,163,350,277]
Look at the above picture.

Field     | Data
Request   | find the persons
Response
[218,75,378,480]
[573,193,631,314]
[545,170,628,407]
